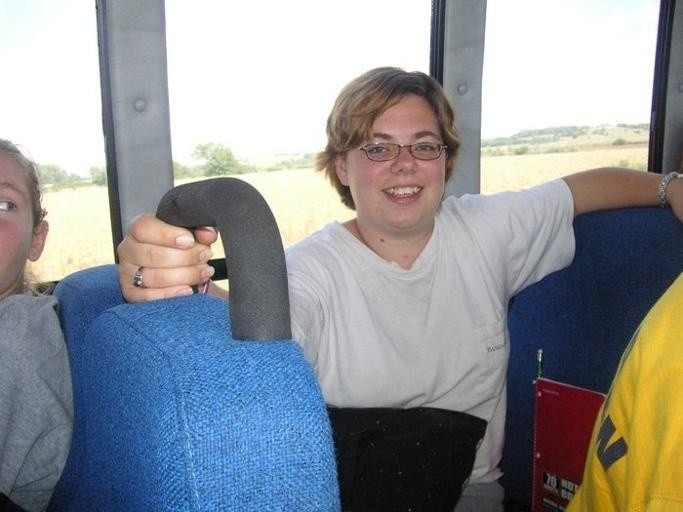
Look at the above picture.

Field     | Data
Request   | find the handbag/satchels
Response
[328,407,488,512]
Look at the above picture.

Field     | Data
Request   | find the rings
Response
[134,266,144,287]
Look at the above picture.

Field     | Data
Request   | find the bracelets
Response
[657,170,679,205]
[202,280,211,295]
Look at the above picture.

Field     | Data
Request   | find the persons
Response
[0,140,74,511]
[566,272,683,509]
[117,66,683,511]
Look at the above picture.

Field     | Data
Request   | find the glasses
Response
[353,143,447,162]
